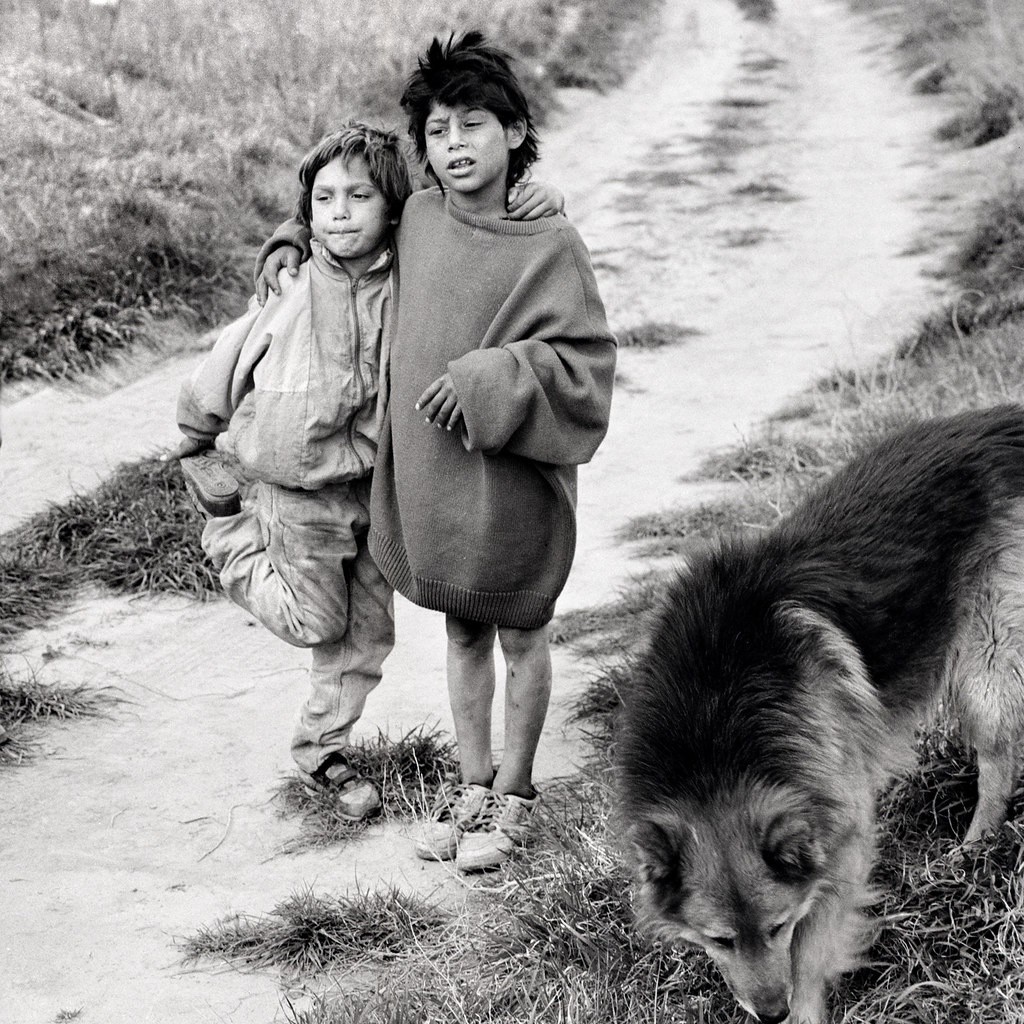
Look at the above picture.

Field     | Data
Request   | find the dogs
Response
[605,402,1023,1023]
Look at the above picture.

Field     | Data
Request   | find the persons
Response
[159,116,412,823]
[253,31,617,872]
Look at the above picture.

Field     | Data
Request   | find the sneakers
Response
[178,443,242,516]
[298,758,382,821]
[415,767,548,873]
